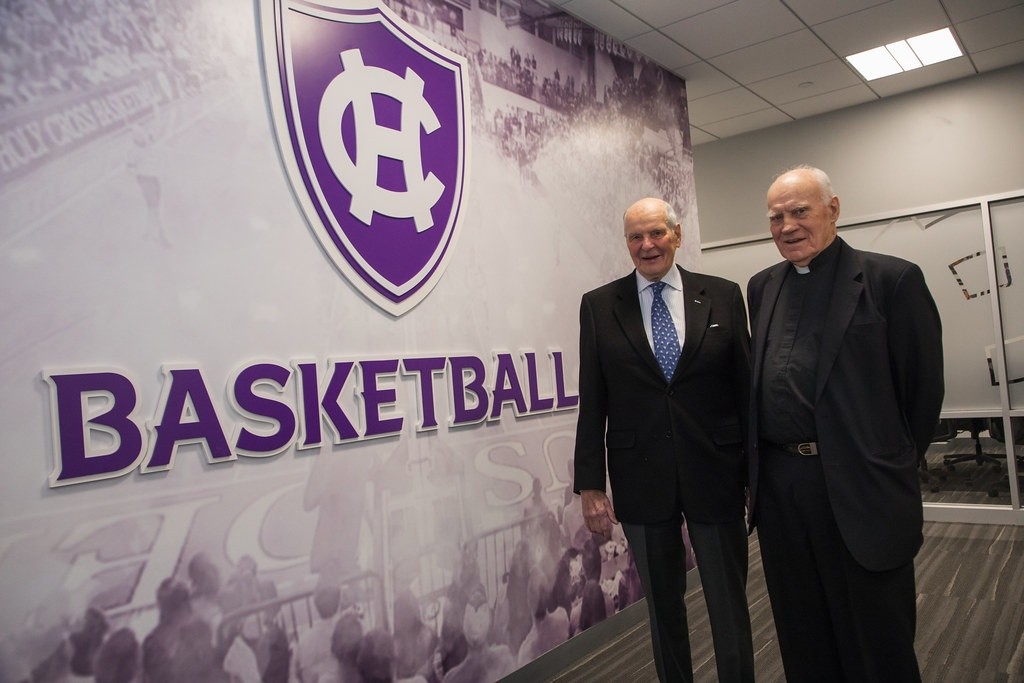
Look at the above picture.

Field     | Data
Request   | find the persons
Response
[385,0,698,219]
[743,166,946,683]
[21,472,694,682]
[0,1,227,108]
[571,198,753,682]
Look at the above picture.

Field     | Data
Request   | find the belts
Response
[760,440,819,458]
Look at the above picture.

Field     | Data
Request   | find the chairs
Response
[942,418,1024,472]
[917,419,957,494]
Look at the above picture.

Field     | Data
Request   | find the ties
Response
[648,282,681,384]
